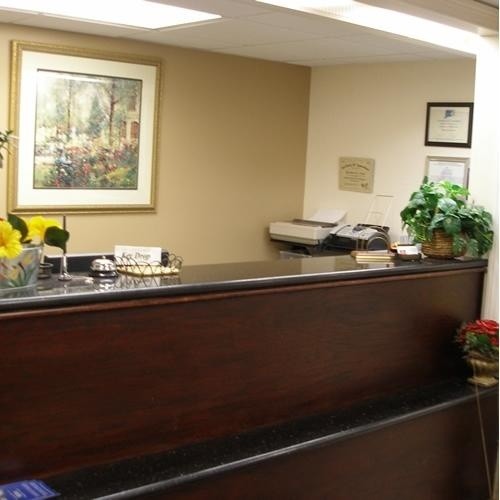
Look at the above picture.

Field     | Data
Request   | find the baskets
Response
[421,225,470,257]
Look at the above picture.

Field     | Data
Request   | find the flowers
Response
[0,209,71,261]
[456,317,499,357]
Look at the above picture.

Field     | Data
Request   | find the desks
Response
[0,250,490,484]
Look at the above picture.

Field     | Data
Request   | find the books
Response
[356,258,394,264]
[351,250,395,258]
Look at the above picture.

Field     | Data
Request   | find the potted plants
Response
[400,179,494,261]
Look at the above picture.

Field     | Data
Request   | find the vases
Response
[0,241,45,293]
[467,348,499,387]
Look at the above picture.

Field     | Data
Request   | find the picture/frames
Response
[6,38,165,216]
[423,99,475,149]
[423,153,471,198]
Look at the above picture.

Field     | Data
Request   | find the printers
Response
[269,218,339,245]
[323,223,391,253]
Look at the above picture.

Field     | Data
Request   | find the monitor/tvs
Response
[44,252,169,274]
